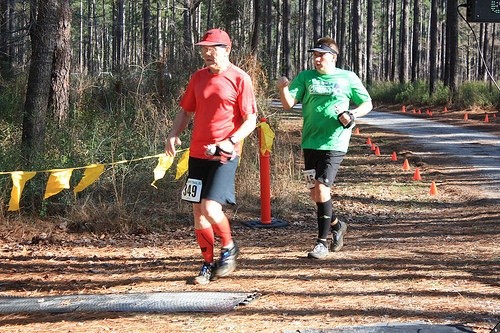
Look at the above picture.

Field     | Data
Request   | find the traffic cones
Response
[370,143,376,151]
[429,181,437,196]
[390,151,398,162]
[426,109,432,116]
[402,158,410,170]
[444,107,448,112]
[412,168,422,181]
[374,146,380,156]
[355,127,360,135]
[401,106,406,112]
[365,138,371,145]
[418,109,422,114]
[412,109,416,113]
[464,112,469,121]
[484,114,489,123]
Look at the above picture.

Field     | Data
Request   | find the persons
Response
[277,37,373,261]
[164,28,258,285]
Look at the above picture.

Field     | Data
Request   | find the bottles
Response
[203,143,236,160]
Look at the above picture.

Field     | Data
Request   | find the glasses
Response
[199,46,227,54]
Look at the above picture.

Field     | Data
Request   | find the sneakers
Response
[330,221,347,252]
[193,261,217,285]
[308,241,328,260]
[216,242,239,277]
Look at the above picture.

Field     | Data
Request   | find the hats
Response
[194,29,231,48]
[307,42,337,57]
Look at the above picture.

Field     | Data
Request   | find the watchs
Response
[228,135,238,146]
